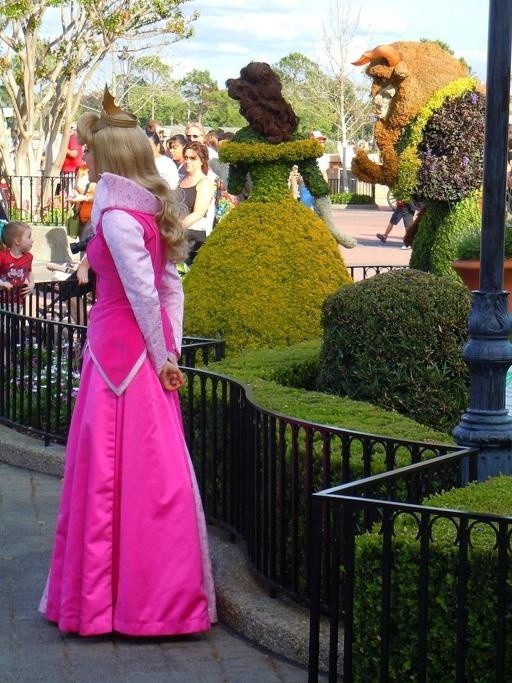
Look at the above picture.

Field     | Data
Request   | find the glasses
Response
[187,134,198,138]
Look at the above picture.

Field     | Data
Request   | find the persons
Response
[291,130,327,214]
[376,200,415,249]
[62,120,235,274]
[0,221,35,367]
[38,112,217,637]
[59,254,95,330]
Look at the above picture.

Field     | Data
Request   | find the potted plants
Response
[452,213,512,311]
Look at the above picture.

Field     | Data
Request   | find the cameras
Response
[70,232,96,254]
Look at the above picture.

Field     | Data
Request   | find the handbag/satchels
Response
[65,216,80,239]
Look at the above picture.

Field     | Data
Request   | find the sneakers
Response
[376,233,386,243]
[400,245,412,249]
